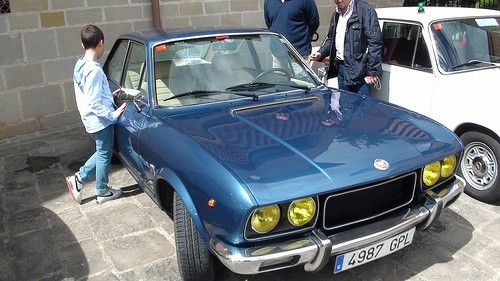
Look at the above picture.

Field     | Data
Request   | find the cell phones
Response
[364,76,370,84]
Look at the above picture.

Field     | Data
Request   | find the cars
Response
[102,27,466,281]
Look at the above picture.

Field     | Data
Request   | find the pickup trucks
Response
[310,3,500,203]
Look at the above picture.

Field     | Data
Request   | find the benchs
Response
[112,62,212,91]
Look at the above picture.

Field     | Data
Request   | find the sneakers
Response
[96,186,122,204]
[65,174,84,204]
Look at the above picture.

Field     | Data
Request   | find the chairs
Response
[208,52,253,101]
[140,60,195,106]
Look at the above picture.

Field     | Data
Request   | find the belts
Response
[338,60,345,65]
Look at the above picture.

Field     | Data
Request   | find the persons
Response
[274,102,312,121]
[65,24,127,204]
[264,0,320,78]
[320,90,362,127]
[310,0,383,96]
